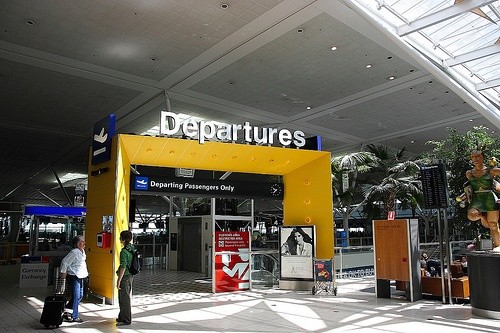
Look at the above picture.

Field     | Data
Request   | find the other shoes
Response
[116,322,125,326]
[116,318,121,322]
[69,317,83,322]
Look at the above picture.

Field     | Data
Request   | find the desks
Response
[466,251,500,320]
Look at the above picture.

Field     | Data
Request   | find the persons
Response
[281,228,313,257]
[18,230,26,241]
[59,235,88,322]
[453,256,468,276]
[115,231,135,326]
[466,149,500,246]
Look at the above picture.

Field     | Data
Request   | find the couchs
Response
[396,259,469,303]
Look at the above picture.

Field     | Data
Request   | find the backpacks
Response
[122,246,141,274]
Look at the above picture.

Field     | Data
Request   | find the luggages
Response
[40,277,66,329]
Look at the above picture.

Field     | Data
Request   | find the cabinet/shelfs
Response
[371,219,421,303]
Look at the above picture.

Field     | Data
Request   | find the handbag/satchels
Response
[450,261,463,278]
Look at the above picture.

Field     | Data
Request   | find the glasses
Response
[120,237,123,240]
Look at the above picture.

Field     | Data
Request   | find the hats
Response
[421,253,427,258]
